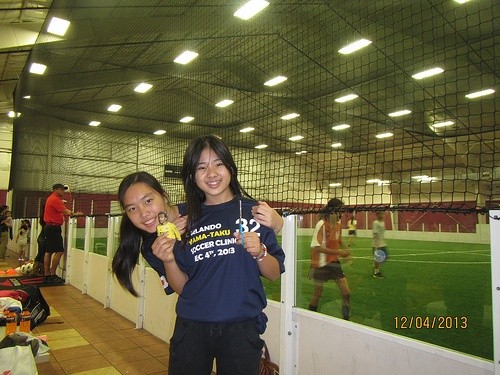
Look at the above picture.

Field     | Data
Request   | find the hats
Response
[375,205,387,211]
[54,184,68,190]
[327,198,346,212]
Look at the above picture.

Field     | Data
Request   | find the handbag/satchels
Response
[259,343,279,375]
[0,285,50,329]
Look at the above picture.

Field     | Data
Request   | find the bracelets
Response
[252,243,267,261]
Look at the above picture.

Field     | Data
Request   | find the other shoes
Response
[45,275,65,285]
[373,272,383,278]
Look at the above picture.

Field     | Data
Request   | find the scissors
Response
[240,199,244,247]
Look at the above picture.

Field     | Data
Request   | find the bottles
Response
[5,309,17,335]
[20,308,31,333]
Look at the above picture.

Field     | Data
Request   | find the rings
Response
[180,216,185,221]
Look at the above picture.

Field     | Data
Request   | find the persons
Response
[338,216,343,249]
[31,196,47,278]
[308,198,350,320]
[157,212,181,241]
[372,210,388,279]
[152,134,285,375]
[44,184,83,284]
[0,204,13,260]
[347,216,358,246]
[16,220,30,261]
[111,171,283,298]
